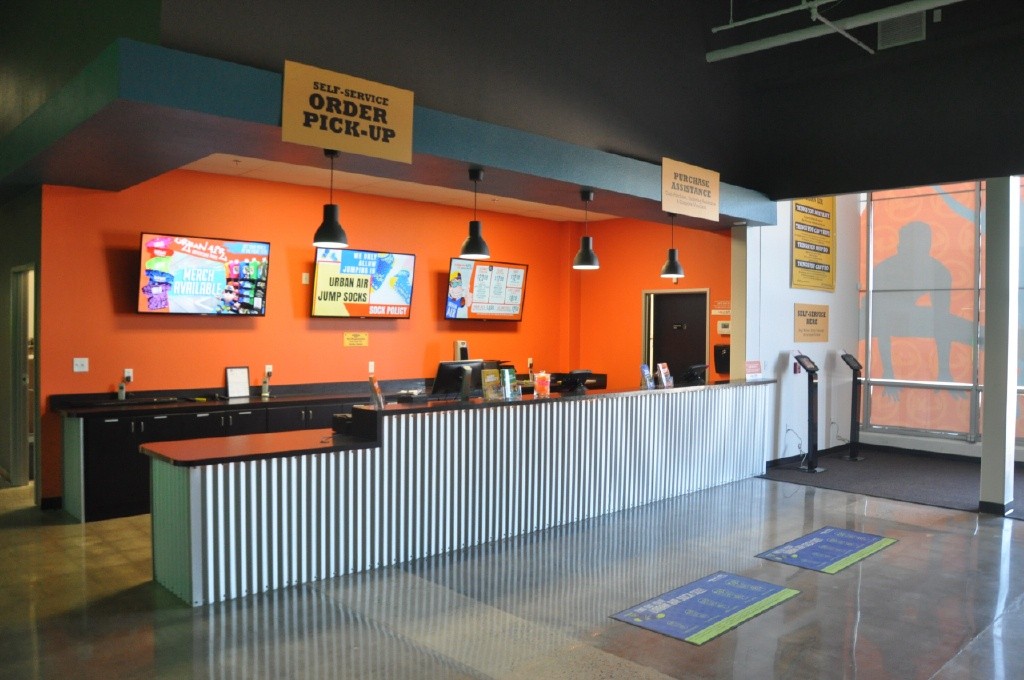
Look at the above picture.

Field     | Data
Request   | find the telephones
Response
[261,377,270,397]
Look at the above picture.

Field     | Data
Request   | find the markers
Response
[188,397,207,401]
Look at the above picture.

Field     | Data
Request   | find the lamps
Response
[312,148,349,249]
[458,169,492,261]
[659,213,684,280]
[572,191,599,270]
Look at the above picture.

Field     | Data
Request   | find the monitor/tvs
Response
[432,359,485,401]
[445,257,528,321]
[135,231,270,316]
[311,248,416,320]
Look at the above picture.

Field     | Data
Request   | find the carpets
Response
[758,443,1024,523]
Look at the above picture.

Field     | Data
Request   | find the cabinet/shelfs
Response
[265,403,344,432]
[62,414,181,522]
[179,407,266,436]
